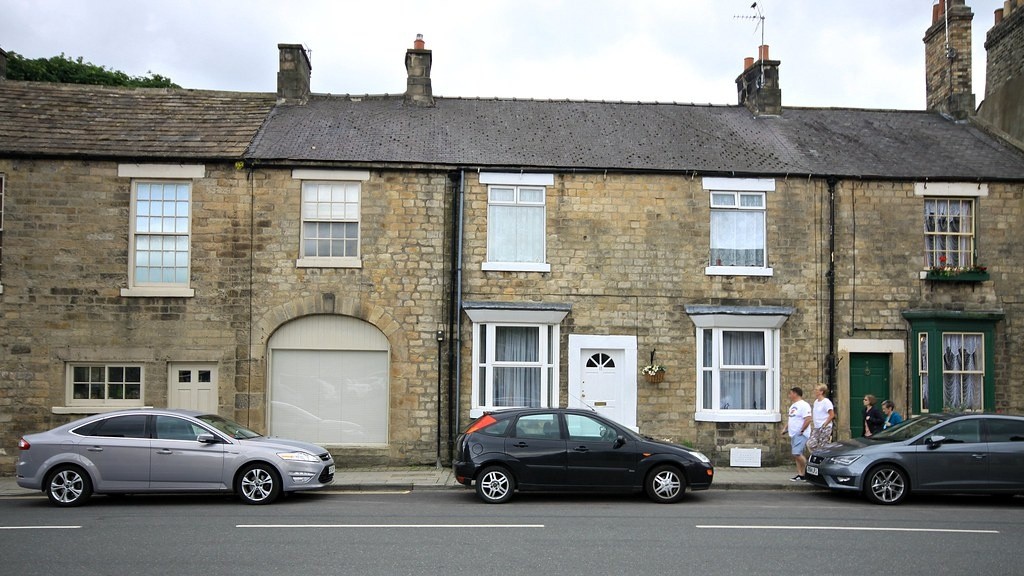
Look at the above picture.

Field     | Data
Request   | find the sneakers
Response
[789,475,807,482]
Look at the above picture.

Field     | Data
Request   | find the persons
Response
[781,386,812,481]
[806,383,834,453]
[861,395,884,438]
[882,400,902,429]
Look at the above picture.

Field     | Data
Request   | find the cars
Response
[15,410,337,504]
[806,411,1024,504]
[450,408,714,504]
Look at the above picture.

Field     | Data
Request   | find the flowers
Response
[641,365,667,377]
[928,256,990,275]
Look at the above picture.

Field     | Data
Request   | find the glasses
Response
[863,399,867,401]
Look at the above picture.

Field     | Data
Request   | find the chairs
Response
[544,423,552,434]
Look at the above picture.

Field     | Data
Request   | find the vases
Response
[644,371,664,383]
[926,270,990,281]
